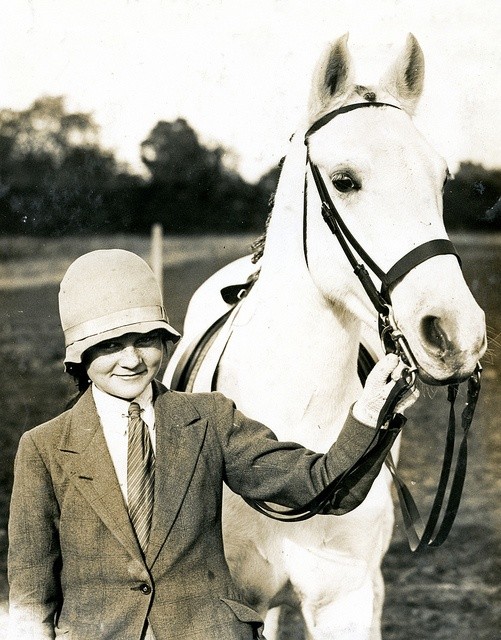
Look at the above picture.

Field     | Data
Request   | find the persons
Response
[8,249,420,639]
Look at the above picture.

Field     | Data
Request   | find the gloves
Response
[353,353,421,430]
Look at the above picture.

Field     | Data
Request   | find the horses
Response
[162,32,501,640]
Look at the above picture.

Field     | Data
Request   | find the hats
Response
[57,247,182,372]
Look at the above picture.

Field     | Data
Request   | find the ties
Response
[124,401,156,557]
